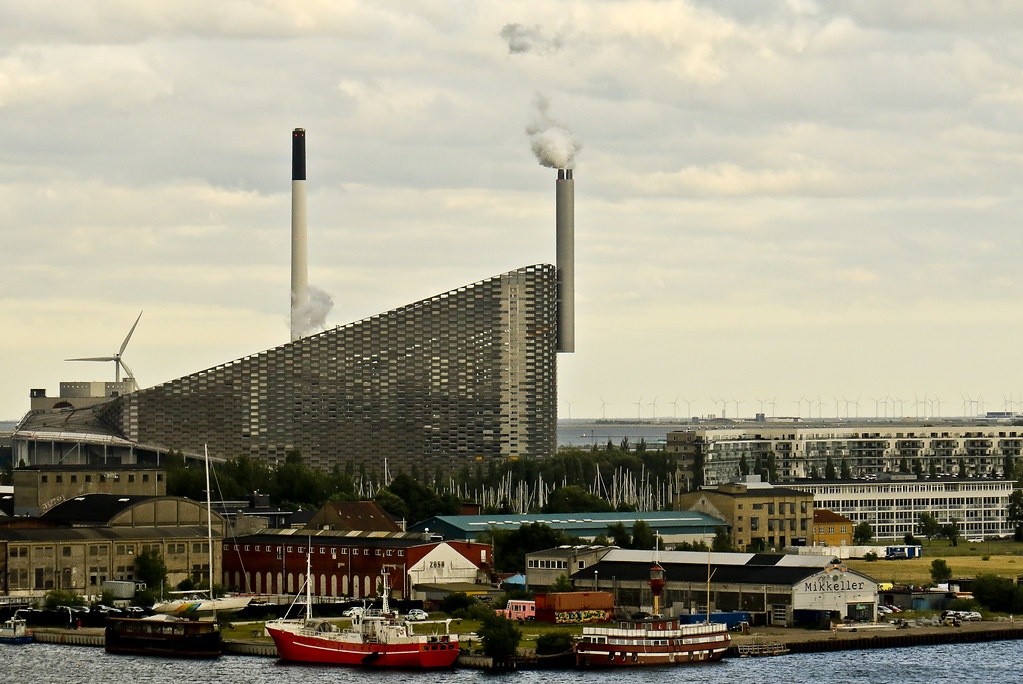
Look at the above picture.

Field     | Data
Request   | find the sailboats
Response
[152,443,254,614]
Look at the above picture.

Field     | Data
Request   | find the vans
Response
[885,552,906,560]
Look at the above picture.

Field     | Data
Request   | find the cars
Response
[849,472,1008,481]
[55,604,144,613]
[941,610,982,620]
[343,607,363,617]
[967,536,984,543]
[405,609,428,621]
[878,605,902,613]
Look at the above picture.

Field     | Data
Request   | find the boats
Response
[571,531,732,667]
[265,535,460,669]
[0,616,34,644]
[105,617,224,660]
